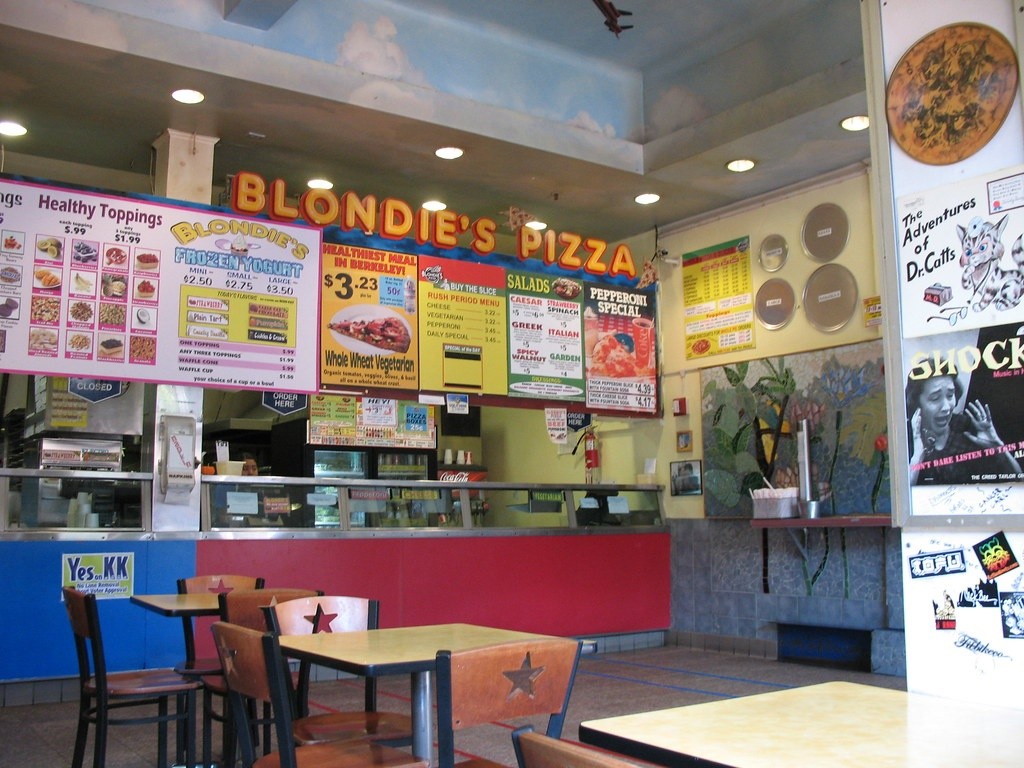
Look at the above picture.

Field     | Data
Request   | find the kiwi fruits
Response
[35,238,62,258]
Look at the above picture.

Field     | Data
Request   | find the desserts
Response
[100,338,123,354]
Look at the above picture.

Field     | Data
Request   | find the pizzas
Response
[326,316,410,353]
[590,335,652,377]
[130,336,156,360]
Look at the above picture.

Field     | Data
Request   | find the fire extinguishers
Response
[570,426,601,468]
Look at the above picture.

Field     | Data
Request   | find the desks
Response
[131,594,226,768]
[577,681,909,768]
[274,623,597,768]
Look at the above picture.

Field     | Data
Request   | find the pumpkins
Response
[202,463,215,475]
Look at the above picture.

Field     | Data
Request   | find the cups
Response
[632,318,655,367]
[66,493,99,529]
[444,449,473,465]
[584,317,599,358]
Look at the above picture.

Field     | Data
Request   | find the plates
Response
[33,271,61,288]
[329,303,412,356]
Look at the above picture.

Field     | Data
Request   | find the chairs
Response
[64,576,669,768]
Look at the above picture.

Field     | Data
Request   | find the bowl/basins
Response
[213,461,246,475]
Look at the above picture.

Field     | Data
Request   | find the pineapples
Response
[102,275,114,296]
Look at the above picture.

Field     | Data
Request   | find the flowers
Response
[768,356,888,512]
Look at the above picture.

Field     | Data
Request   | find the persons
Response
[212,453,258,525]
[905,359,1024,487]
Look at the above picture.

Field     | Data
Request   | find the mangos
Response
[36,269,61,286]
